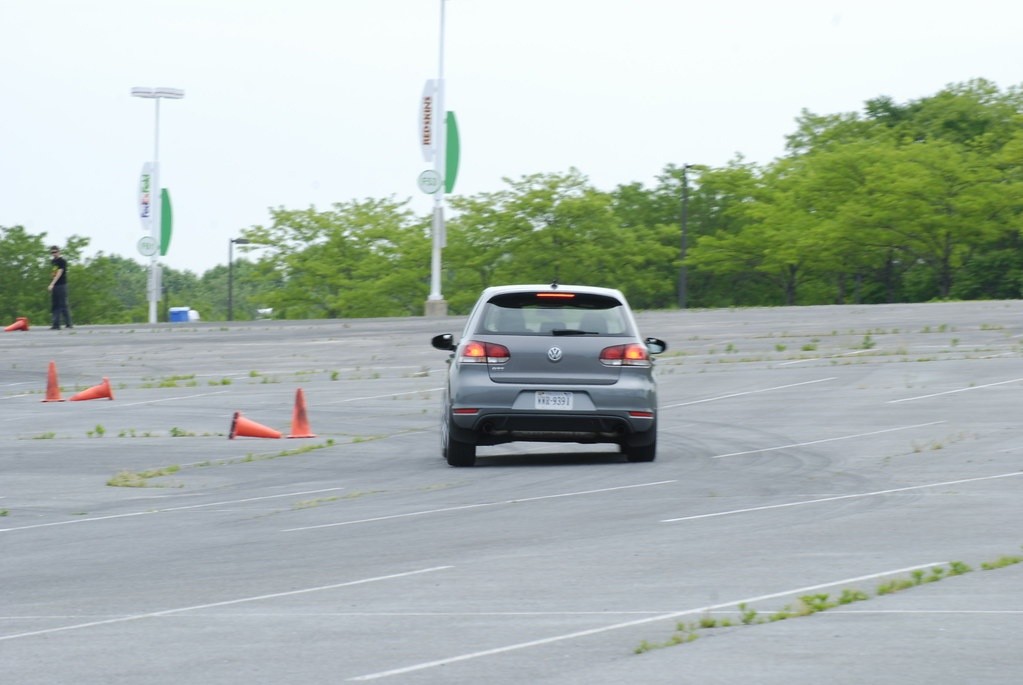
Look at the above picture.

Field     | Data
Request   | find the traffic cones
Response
[68,376,114,401]
[41,361,66,402]
[3,317,28,331]
[284,387,317,439]
[228,411,282,440]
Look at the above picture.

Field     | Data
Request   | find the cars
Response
[429,281,667,469]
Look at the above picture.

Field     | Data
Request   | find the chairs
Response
[580,313,608,333]
[495,309,525,332]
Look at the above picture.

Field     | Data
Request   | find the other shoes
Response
[65,325,73,328]
[51,327,61,330]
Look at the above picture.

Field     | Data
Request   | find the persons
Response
[47,246,72,330]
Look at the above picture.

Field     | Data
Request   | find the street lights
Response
[678,164,709,309]
[131,86,185,323]
[227,238,251,321]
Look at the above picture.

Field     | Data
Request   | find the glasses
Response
[51,251,58,254]
